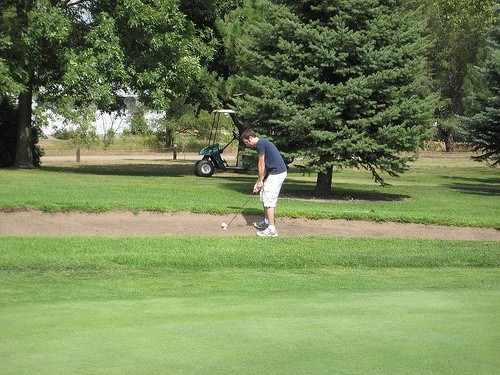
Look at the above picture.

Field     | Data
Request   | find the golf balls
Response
[221,223,226,227]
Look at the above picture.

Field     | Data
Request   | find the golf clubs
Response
[223,192,255,230]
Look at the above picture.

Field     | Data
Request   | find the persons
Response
[240,129,288,237]
